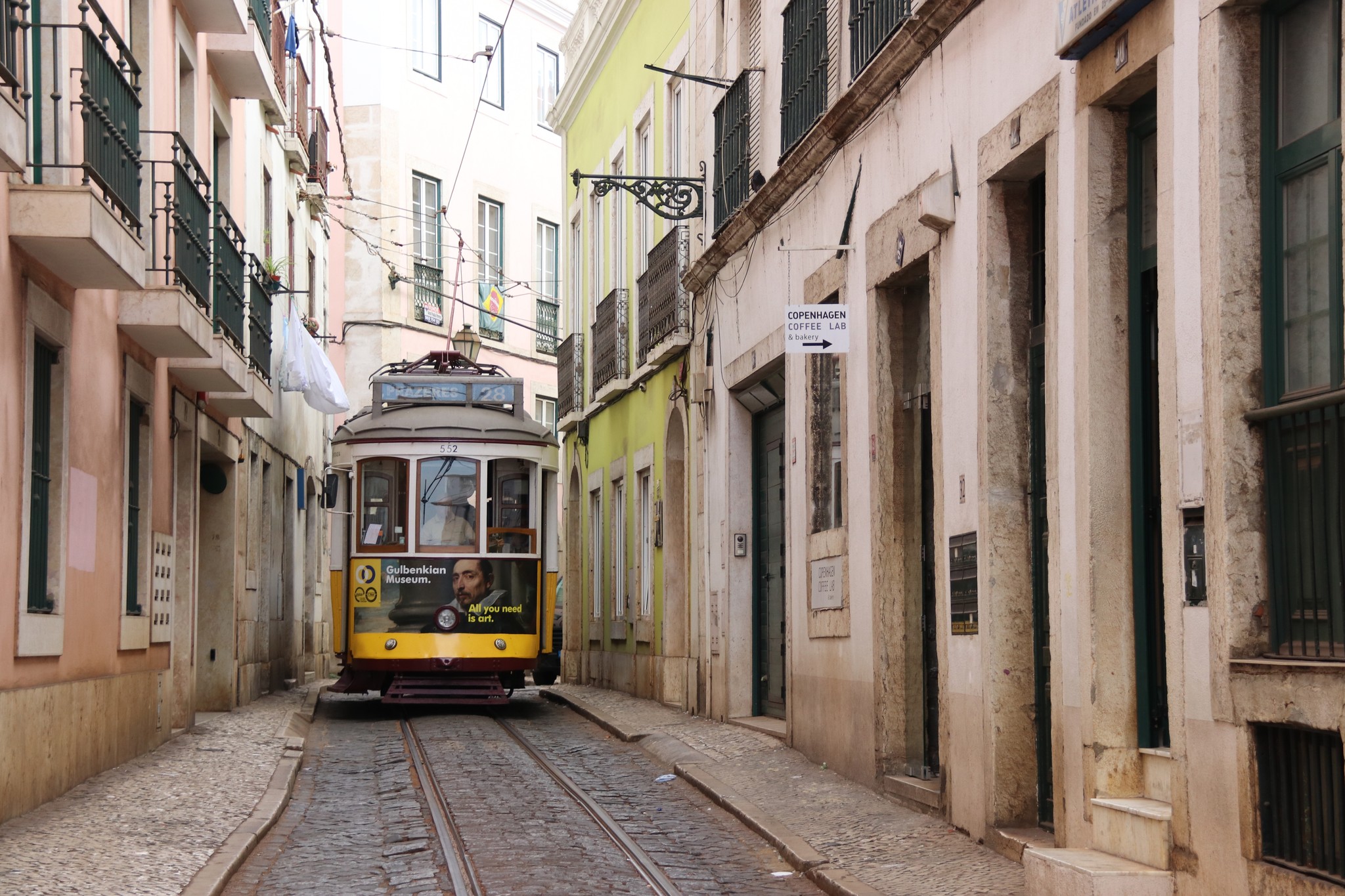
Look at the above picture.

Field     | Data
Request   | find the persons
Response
[420,559,533,634]
[421,492,476,545]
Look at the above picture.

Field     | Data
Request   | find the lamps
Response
[451,323,481,369]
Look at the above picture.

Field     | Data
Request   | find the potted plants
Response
[262,255,296,289]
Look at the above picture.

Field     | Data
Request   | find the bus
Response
[312,234,561,706]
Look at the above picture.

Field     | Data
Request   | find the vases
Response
[273,280,281,290]
